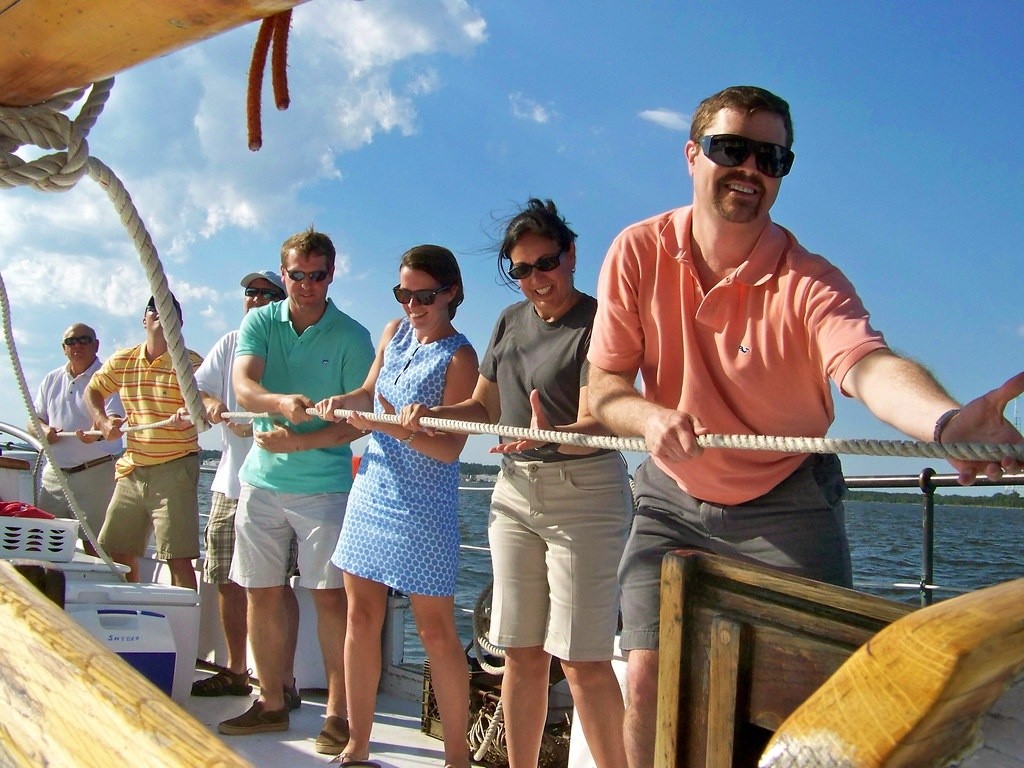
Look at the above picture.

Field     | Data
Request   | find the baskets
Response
[0,516,81,562]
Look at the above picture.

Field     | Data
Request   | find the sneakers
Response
[218,699,290,734]
[315,716,350,754]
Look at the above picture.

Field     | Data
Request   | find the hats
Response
[147,293,180,306]
[240,270,288,297]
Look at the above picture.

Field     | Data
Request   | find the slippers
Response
[328,753,381,768]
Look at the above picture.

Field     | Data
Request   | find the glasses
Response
[393,283,451,305]
[507,246,564,279]
[64,336,93,345]
[145,305,156,318]
[285,267,329,282]
[245,288,284,300]
[696,134,794,179]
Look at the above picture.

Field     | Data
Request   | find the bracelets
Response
[934,407,960,441]
[538,443,561,454]
[402,432,417,444]
[97,435,103,441]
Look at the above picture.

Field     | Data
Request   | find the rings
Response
[243,433,245,436]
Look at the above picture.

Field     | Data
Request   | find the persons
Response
[314,245,480,768]
[401,199,634,768]
[218,232,375,751]
[84,289,205,591]
[192,270,303,712]
[586,86,1024,768]
[27,323,126,557]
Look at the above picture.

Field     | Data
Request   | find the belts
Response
[58,454,120,474]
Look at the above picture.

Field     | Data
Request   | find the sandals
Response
[191,667,253,697]
[283,678,302,709]
